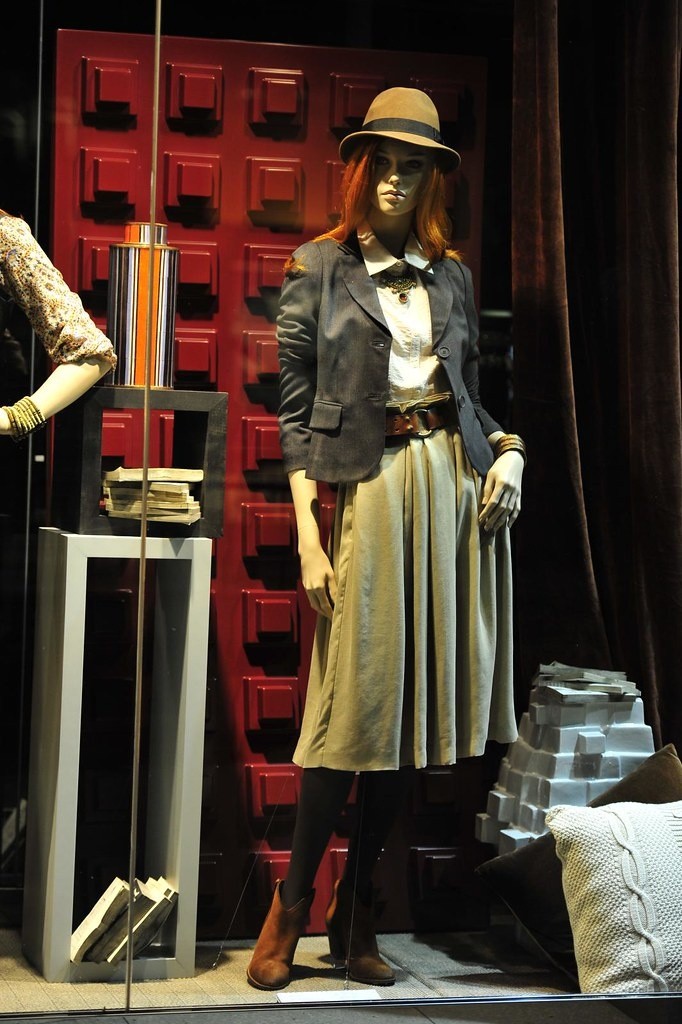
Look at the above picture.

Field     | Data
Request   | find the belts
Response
[385,403,458,436]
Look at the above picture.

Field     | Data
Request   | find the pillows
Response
[473,744,682,987]
[543,800,682,994]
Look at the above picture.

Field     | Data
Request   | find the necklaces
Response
[371,265,419,303]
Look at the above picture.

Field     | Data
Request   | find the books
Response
[100,467,204,525]
[532,661,642,705]
[69,876,179,966]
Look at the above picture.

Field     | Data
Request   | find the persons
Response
[0,212,117,437]
[245,87,525,989]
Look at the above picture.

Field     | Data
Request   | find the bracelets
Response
[2,394,47,442]
[494,433,527,468]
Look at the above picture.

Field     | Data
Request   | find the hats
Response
[338,87,462,175]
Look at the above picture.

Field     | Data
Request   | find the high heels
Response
[325,878,396,984]
[246,879,316,990]
